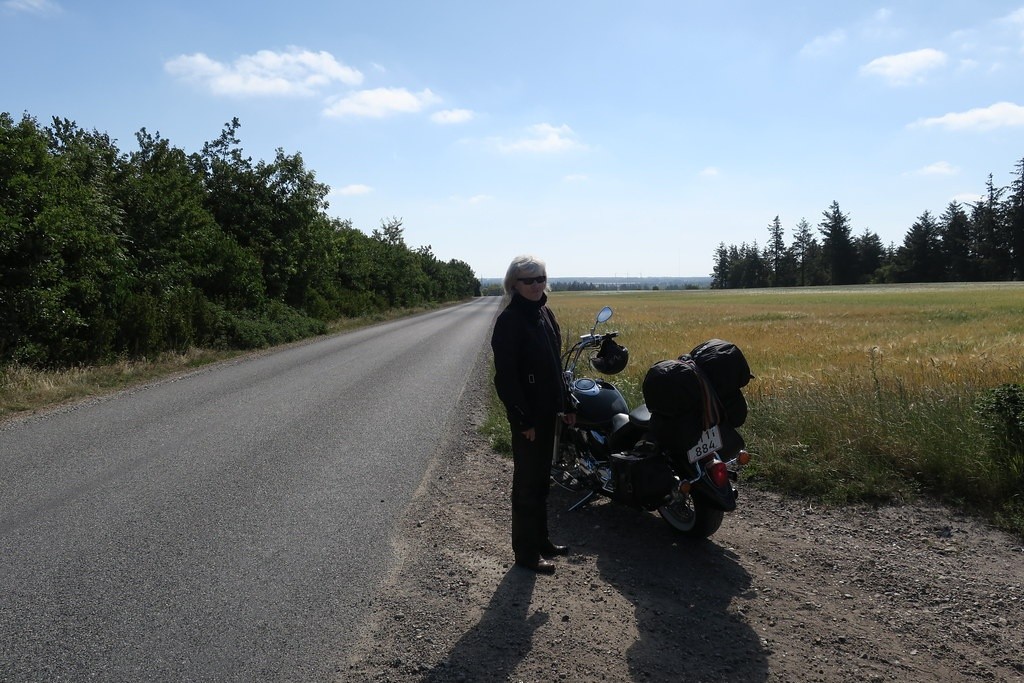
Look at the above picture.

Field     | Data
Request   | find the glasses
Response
[517,276,547,285]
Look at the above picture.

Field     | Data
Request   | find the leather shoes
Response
[514,546,556,574]
[535,539,570,556]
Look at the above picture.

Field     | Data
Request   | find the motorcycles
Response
[544,302,754,544]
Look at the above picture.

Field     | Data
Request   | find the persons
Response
[490,255,569,572]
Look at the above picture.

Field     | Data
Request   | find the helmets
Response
[589,340,629,374]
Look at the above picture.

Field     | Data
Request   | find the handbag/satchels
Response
[642,338,756,457]
